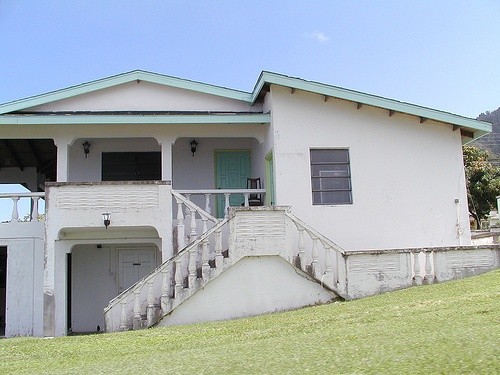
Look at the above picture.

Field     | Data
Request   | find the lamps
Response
[82,140,91,158]
[102,213,111,230]
[189,139,198,156]
